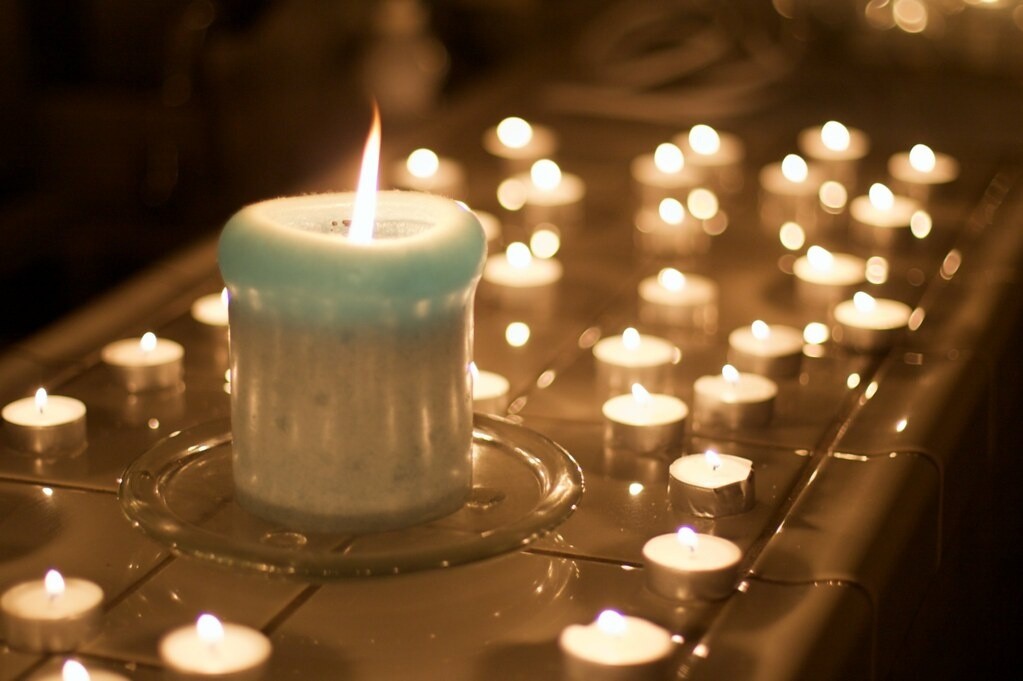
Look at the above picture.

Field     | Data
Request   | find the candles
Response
[0,105,965,681]
